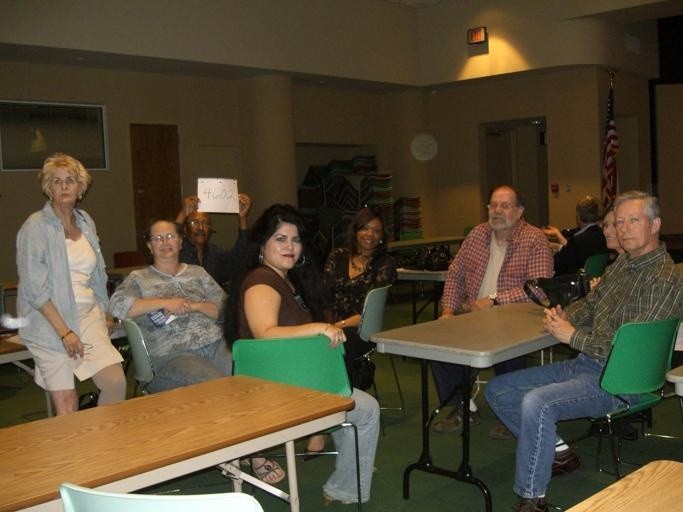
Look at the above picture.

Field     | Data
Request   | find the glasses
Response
[486,203,519,209]
[188,219,209,225]
[152,234,180,241]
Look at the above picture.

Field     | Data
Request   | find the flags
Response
[597,80,621,214]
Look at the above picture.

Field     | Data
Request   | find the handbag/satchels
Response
[523,275,585,307]
[425,243,452,271]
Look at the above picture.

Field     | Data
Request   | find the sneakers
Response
[551,448,580,478]
[513,499,552,512]
[432,405,479,432]
[488,420,514,440]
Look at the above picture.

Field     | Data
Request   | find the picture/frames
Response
[0,98,110,173]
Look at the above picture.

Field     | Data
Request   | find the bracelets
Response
[339,319,346,329]
[60,330,73,341]
[323,322,331,336]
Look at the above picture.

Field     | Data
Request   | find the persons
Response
[581,201,626,296]
[429,183,555,440]
[107,215,287,487]
[302,205,398,463]
[481,188,682,511]
[174,191,252,290]
[226,203,381,508]
[14,151,127,419]
[538,194,608,273]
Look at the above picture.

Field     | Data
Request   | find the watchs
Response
[485,293,499,307]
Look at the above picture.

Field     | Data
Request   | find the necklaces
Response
[349,252,365,274]
[61,224,77,235]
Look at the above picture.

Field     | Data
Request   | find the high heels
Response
[303,448,324,461]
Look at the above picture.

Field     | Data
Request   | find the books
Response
[357,167,425,244]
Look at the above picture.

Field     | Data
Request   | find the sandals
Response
[251,456,284,484]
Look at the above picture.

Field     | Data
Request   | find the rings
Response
[333,336,339,342]
